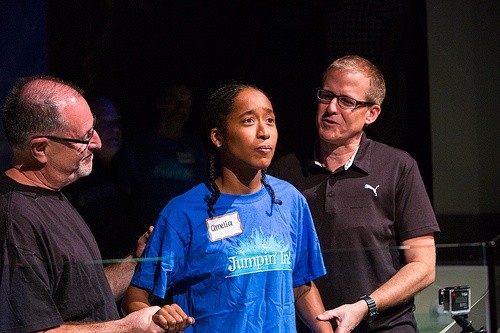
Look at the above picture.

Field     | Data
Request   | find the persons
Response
[0,74,167,333]
[61,85,221,267]
[61,79,224,259]
[266,55,440,333]
[123,82,333,333]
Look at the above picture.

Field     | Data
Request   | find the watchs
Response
[358,295,380,320]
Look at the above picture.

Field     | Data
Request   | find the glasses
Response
[317,88,375,109]
[28,115,97,144]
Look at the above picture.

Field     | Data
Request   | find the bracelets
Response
[131,252,140,262]
